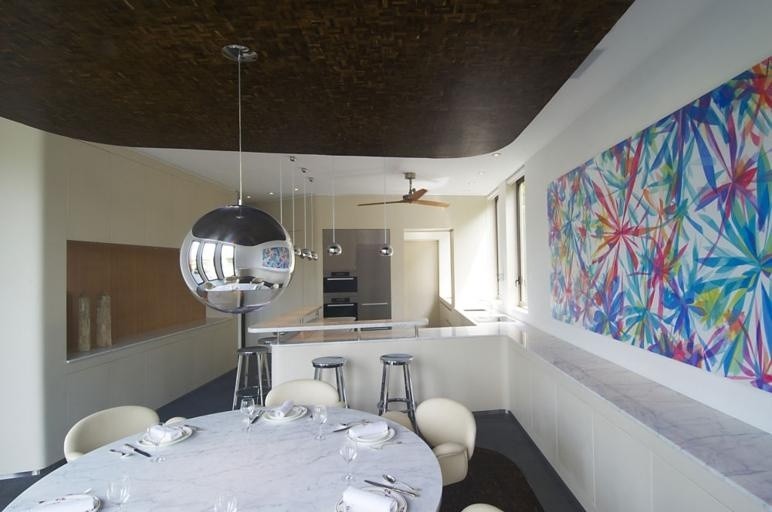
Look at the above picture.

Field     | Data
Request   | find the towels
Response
[349,420,388,437]
[148,423,182,441]
[342,487,398,512]
[274,398,295,417]
[32,498,93,512]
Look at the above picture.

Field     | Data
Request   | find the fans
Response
[357,172,449,209]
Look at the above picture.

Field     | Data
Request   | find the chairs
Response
[462,502,502,512]
[379,397,477,487]
[62,404,159,462]
[264,378,340,407]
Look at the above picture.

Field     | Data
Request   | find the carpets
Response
[438,447,545,512]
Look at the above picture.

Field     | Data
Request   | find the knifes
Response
[332,420,369,432]
[364,479,417,497]
[124,443,152,457]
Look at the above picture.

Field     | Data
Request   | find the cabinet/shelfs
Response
[508,321,772,512]
[247,298,508,416]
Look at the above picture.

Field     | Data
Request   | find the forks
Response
[184,424,206,431]
[369,441,403,450]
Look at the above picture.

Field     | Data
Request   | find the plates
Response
[345,423,396,444]
[143,426,187,445]
[28,494,101,512]
[336,486,408,511]
[355,429,389,442]
[136,424,193,448]
[268,406,302,419]
[262,405,308,422]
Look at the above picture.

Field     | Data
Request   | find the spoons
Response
[110,449,135,456]
[382,473,423,491]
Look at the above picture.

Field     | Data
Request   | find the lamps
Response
[309,177,319,260]
[293,182,302,256]
[301,168,313,262]
[377,175,394,256]
[179,43,296,315]
[327,182,342,256]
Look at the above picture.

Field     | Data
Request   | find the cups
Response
[105,473,131,511]
[214,495,238,511]
[311,402,329,440]
[240,397,255,432]
[339,440,358,482]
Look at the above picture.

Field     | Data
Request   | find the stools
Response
[311,356,350,409]
[231,346,271,410]
[257,336,277,385]
[376,353,416,434]
